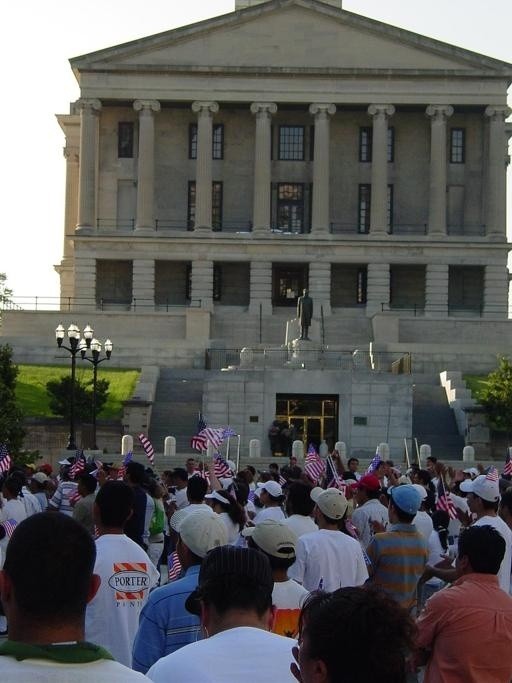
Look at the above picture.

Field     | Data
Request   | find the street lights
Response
[54,325,92,452]
[78,337,113,449]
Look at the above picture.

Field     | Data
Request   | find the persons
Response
[350,474,392,553]
[297,288,313,340]
[242,519,311,640]
[290,584,424,683]
[365,484,430,614]
[0,509,156,683]
[254,444,328,520]
[98,467,266,564]
[287,487,370,592]
[131,510,229,675]
[0,479,26,639]
[414,524,512,683]
[8,454,100,521]
[145,544,299,683]
[84,479,160,669]
[417,510,449,610]
[419,475,511,596]
[269,420,299,456]
[500,487,511,528]
[329,448,481,486]
[449,480,477,569]
[411,481,435,545]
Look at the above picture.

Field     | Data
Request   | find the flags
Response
[67,451,85,480]
[118,450,133,479]
[166,552,182,580]
[502,458,511,478]
[0,444,11,475]
[485,466,497,482]
[435,492,456,519]
[2,517,18,536]
[191,427,236,478]
[136,432,170,497]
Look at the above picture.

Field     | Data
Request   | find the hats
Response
[25,471,51,484]
[459,474,501,502]
[185,544,274,616]
[310,486,349,521]
[169,509,230,560]
[386,484,422,515]
[204,490,231,505]
[461,467,479,476]
[350,474,381,492]
[410,483,428,502]
[58,458,72,465]
[241,518,299,558]
[256,479,283,498]
[170,503,214,532]
